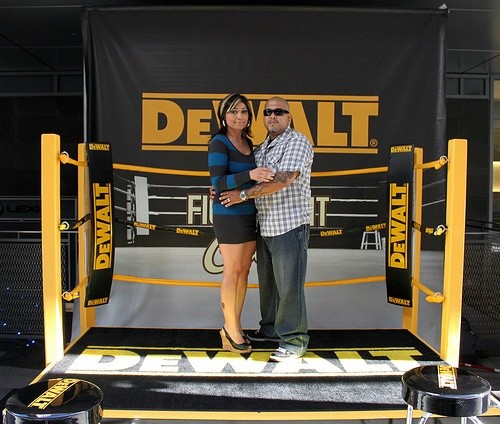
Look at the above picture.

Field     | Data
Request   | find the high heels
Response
[241,333,251,344]
[220,326,252,353]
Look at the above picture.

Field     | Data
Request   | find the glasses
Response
[263,108,289,116]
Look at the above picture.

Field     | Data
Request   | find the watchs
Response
[239,190,248,201]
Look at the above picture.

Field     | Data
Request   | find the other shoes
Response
[269,347,302,361]
[247,330,281,342]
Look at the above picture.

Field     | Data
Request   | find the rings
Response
[227,198,231,202]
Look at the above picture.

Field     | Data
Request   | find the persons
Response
[208,94,275,355]
[210,96,314,362]
